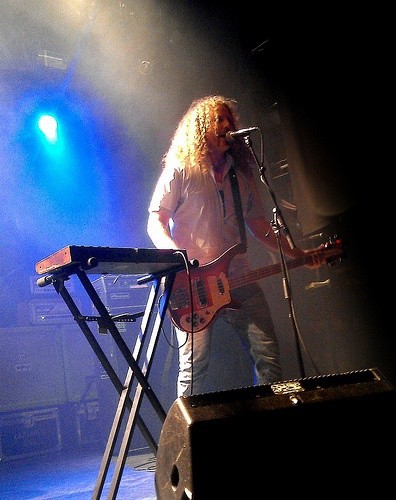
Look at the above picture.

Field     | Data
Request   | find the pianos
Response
[35,245,190,500]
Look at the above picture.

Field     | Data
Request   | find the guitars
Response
[166,234,343,334]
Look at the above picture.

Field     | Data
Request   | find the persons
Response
[146,97,332,399]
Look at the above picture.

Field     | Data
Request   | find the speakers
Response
[0,307,180,457]
[155,367,396,500]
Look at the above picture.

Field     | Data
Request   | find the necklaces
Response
[212,158,227,172]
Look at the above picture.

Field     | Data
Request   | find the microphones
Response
[226,127,258,143]
